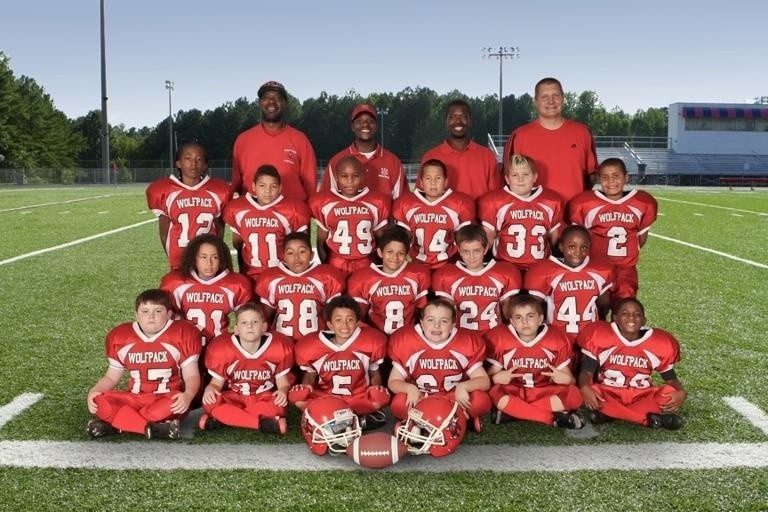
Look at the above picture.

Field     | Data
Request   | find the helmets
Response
[301,396,362,457]
[397,397,467,456]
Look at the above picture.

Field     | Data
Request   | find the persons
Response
[229,81,320,206]
[83,142,690,452]
[416,100,502,202]
[502,78,600,234]
[321,103,412,203]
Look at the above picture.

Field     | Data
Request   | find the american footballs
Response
[344,432,409,469]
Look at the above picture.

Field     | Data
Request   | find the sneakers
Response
[590,414,614,426]
[552,411,586,430]
[463,419,482,434]
[145,418,180,439]
[261,418,287,436]
[359,410,386,433]
[88,418,114,437]
[646,414,682,430]
[199,416,221,432]
[490,412,511,425]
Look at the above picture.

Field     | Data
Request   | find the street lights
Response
[480,46,519,145]
[164,80,175,173]
[375,106,389,147]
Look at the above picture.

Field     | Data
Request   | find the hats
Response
[353,104,378,121]
[257,80,287,98]
